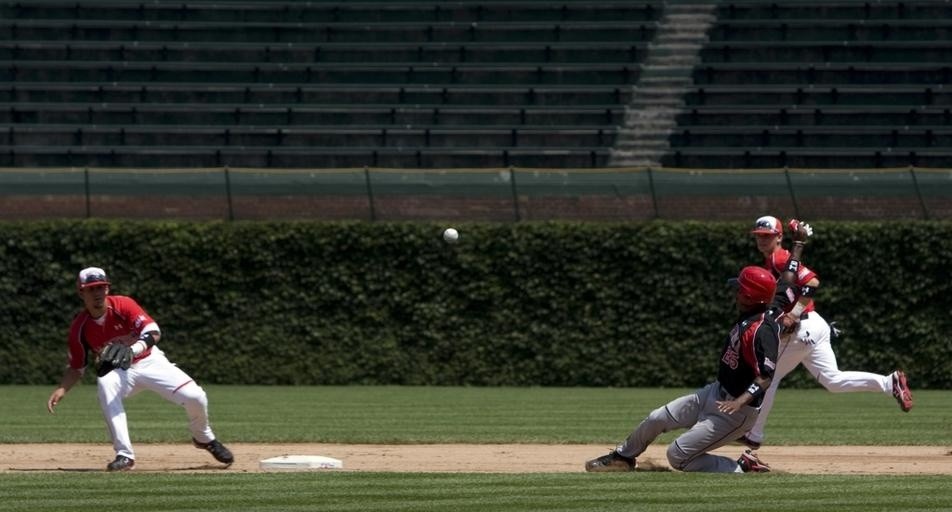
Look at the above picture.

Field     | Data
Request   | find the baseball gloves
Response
[95,341,132,379]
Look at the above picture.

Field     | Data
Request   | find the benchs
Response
[0,0,952,167]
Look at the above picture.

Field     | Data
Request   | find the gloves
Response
[792,221,813,247]
[800,331,815,345]
[831,322,841,338]
[778,312,800,335]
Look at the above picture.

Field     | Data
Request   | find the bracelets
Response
[137,333,155,349]
[781,258,800,274]
[129,340,148,356]
[799,285,817,299]
[744,381,764,398]
[791,300,807,318]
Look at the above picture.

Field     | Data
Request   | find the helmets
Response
[728,266,777,305]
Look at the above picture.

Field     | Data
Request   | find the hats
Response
[750,215,783,234]
[76,266,110,289]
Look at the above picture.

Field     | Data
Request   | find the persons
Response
[585,266,781,474]
[48,266,233,472]
[733,214,913,451]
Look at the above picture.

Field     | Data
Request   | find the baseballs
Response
[444,229,458,241]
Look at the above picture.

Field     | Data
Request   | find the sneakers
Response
[586,450,636,471]
[735,435,760,450]
[107,456,133,471]
[737,449,771,472]
[893,369,913,412]
[192,436,232,462]
